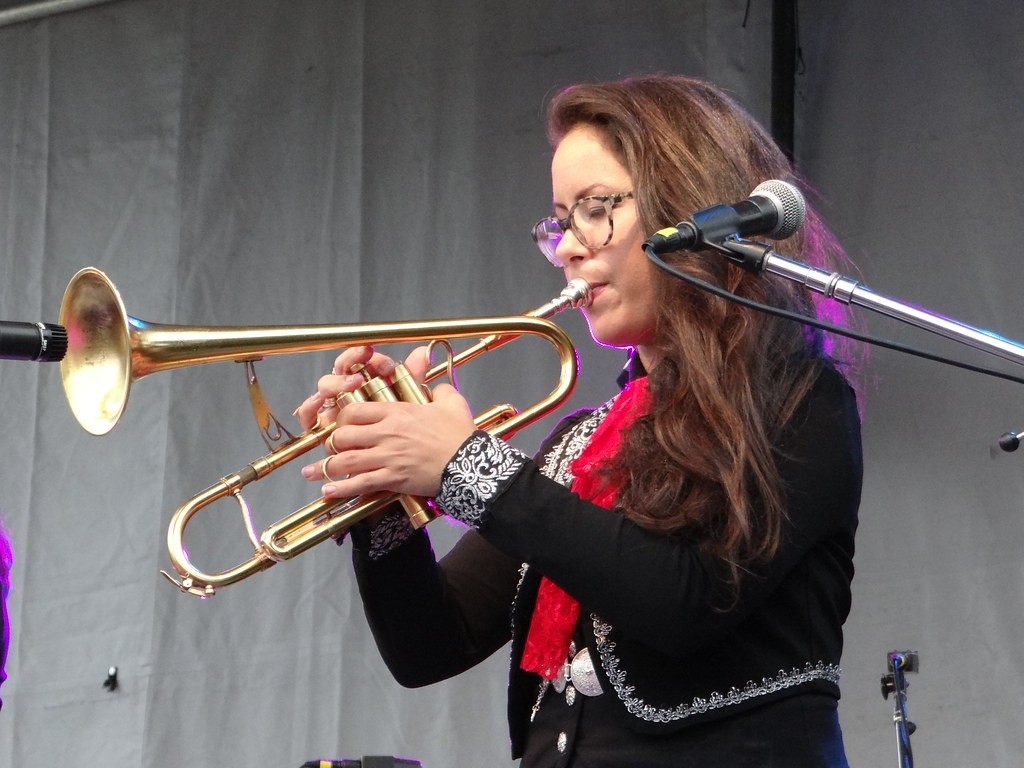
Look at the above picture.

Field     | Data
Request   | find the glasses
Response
[530,192,638,269]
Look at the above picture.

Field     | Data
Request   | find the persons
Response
[298,76,866,768]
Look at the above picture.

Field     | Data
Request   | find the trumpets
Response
[59,267,597,600]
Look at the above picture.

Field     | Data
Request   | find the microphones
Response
[887,650,918,674]
[641,180,806,253]
[0,321,68,362]
[306,756,422,768]
